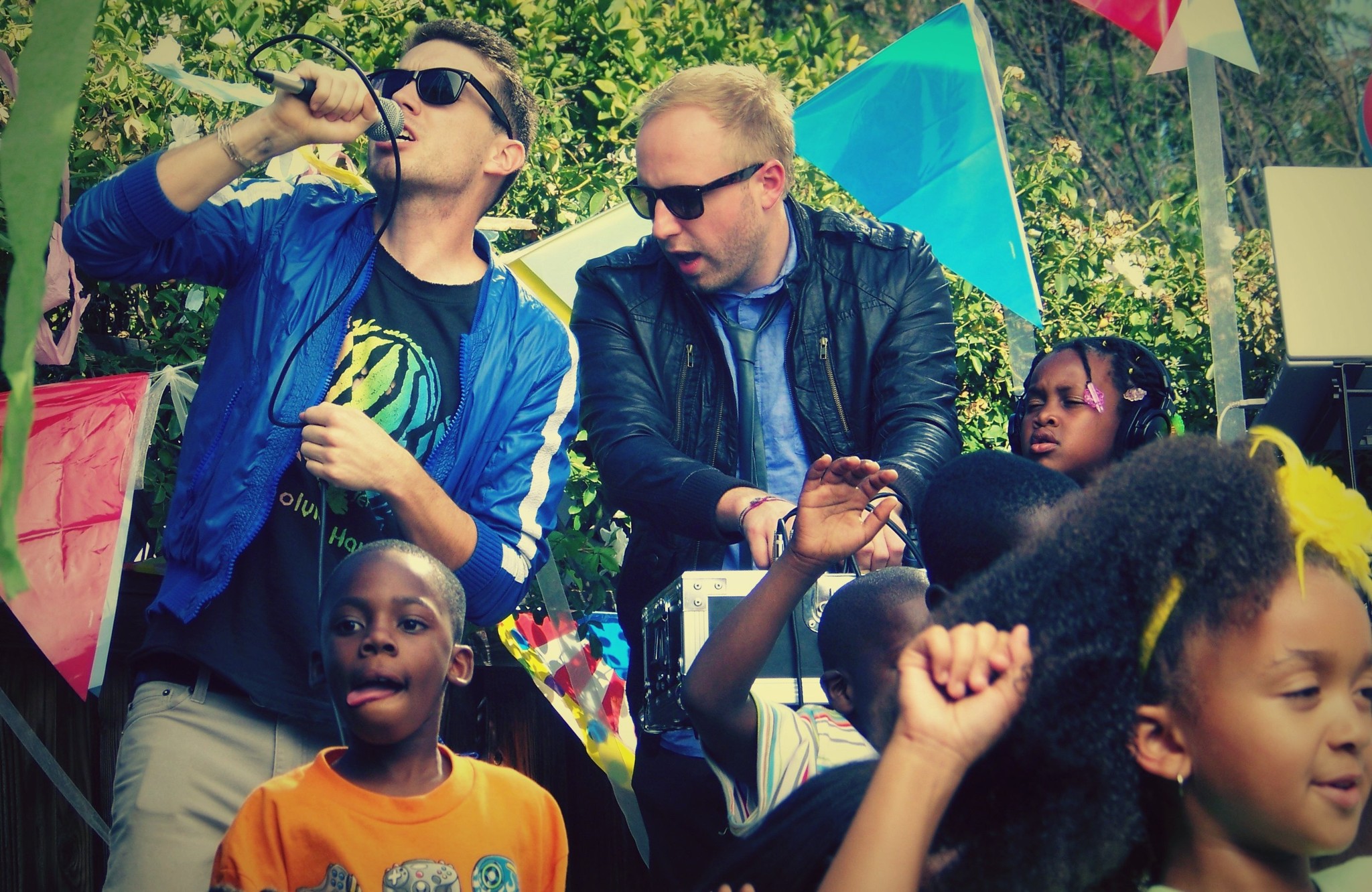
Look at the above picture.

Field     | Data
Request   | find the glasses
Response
[361,69,513,141]
[623,162,768,220]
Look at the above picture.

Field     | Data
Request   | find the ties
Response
[707,289,787,570]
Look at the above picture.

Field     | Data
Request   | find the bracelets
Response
[217,123,259,171]
[738,496,793,538]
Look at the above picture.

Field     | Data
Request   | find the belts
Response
[132,659,240,697]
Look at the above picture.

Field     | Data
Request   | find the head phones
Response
[1008,336,1173,461]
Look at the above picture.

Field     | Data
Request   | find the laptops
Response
[1246,165,1372,460]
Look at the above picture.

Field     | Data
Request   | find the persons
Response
[570,63,963,892]
[209,539,568,892]
[682,333,1372,892]
[60,20,580,892]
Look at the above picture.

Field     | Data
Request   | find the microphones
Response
[253,67,404,141]
